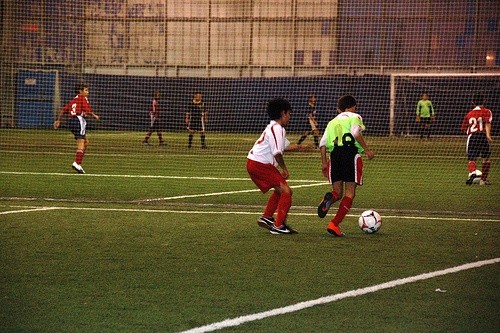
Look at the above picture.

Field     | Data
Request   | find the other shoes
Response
[160,143,166,146]
[202,146,207,149]
[188,145,192,149]
[294,142,299,146]
[143,142,152,146]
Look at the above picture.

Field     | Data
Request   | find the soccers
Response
[469,170,482,184]
[358,210,382,234]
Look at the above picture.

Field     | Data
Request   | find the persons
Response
[185,90,209,149]
[248,97,313,235]
[459,93,494,186]
[141,89,168,146]
[416,92,436,139]
[297,96,319,147]
[53,84,99,174]
[317,95,374,238]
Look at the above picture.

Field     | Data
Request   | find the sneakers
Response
[327,223,346,237]
[480,180,491,185]
[318,192,336,218]
[270,223,298,235]
[257,217,275,230]
[466,172,476,185]
[72,162,86,174]
